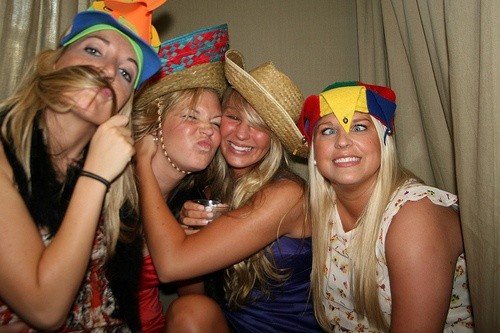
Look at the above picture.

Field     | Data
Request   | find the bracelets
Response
[79,170,111,189]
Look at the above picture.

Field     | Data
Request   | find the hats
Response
[131,23,232,176]
[297,80,397,159]
[57,0,168,93]
[225,47,310,162]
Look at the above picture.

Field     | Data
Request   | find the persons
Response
[0,8,330,333]
[309,81,472,333]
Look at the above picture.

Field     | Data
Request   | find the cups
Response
[193,199,232,230]
